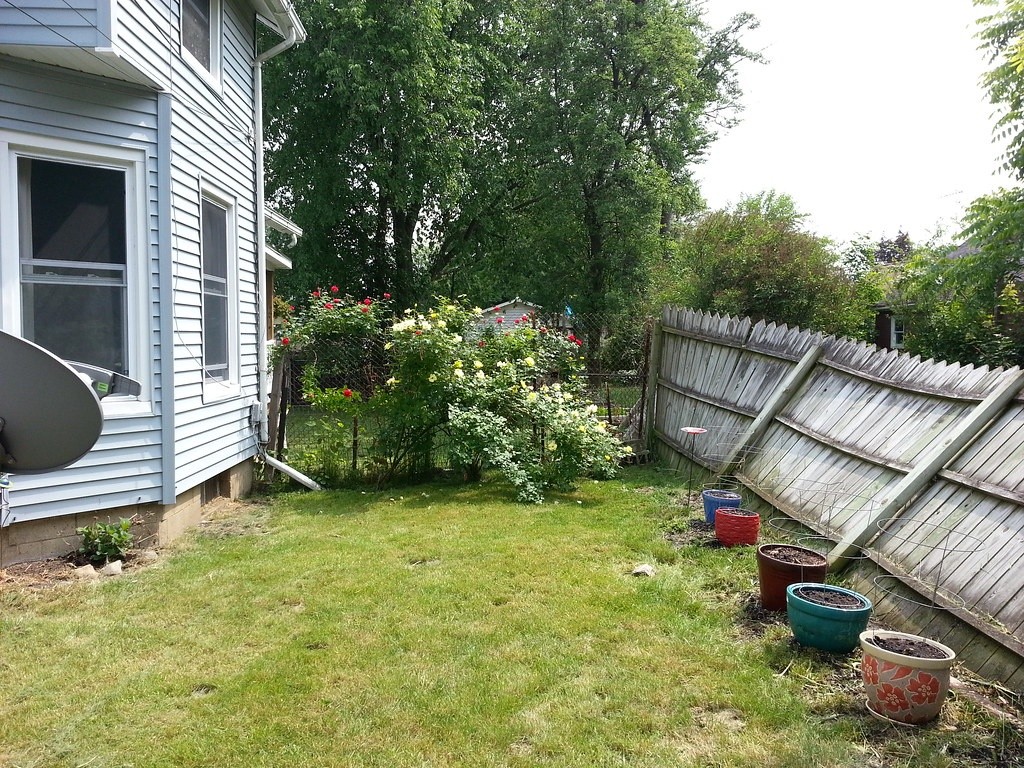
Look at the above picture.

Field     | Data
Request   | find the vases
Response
[858,628,956,727]
[702,489,742,525]
[786,582,872,653]
[715,508,760,546]
[756,543,828,611]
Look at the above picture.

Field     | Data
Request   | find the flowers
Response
[277,285,636,499]
